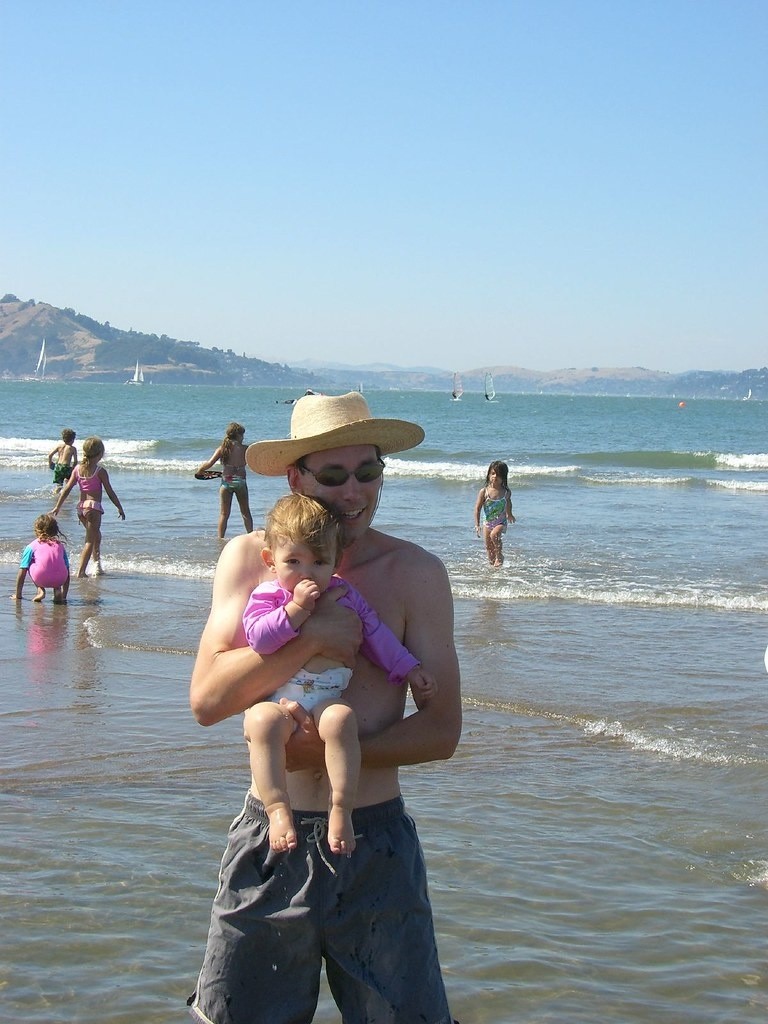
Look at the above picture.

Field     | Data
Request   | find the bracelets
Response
[474,525,482,532]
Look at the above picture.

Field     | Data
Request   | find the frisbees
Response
[195,469,222,479]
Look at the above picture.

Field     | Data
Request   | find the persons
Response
[190,392,464,1023]
[242,493,439,855]
[473,461,517,568]
[48,436,126,578]
[10,515,71,602]
[484,393,489,401]
[197,421,253,539]
[452,391,457,399]
[275,398,297,405]
[48,428,78,495]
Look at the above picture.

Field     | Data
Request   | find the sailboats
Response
[484,371,499,403]
[450,370,464,402]
[124,359,144,386]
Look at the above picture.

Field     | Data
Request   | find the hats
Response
[245,392,425,476]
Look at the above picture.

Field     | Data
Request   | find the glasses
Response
[298,456,385,486]
